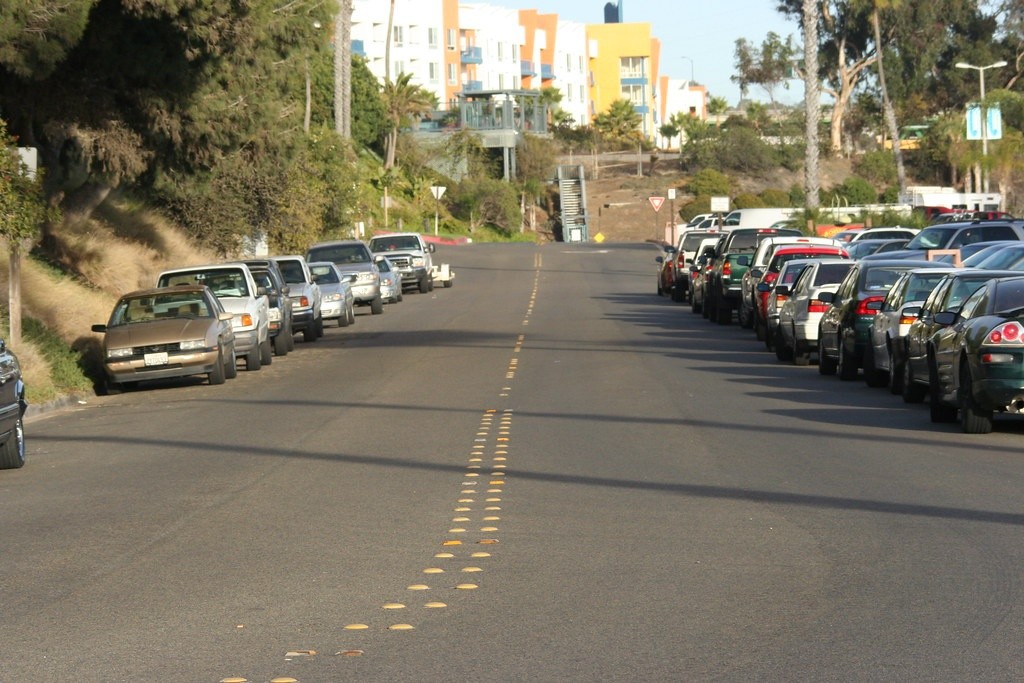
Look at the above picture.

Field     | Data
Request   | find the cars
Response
[924,276,1024,434]
[0,337,27,470]
[91,285,238,395]
[306,262,355,327]
[266,256,323,343]
[737,237,843,330]
[690,248,715,313]
[832,219,1024,272]
[707,234,729,325]
[910,205,955,222]
[675,230,729,293]
[932,213,974,224]
[866,268,987,396]
[817,259,955,381]
[751,244,848,341]
[689,238,720,278]
[776,262,856,364]
[370,232,436,293]
[757,259,855,351]
[974,211,1014,221]
[373,254,403,304]
[654,252,676,300]
[225,260,293,355]
[953,208,974,213]
[902,270,1024,404]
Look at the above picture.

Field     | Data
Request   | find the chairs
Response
[130,268,301,321]
[865,269,971,302]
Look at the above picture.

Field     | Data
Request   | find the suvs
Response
[713,229,802,323]
[303,240,383,315]
[156,262,272,371]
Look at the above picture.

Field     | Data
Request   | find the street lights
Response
[955,60,1008,193]
